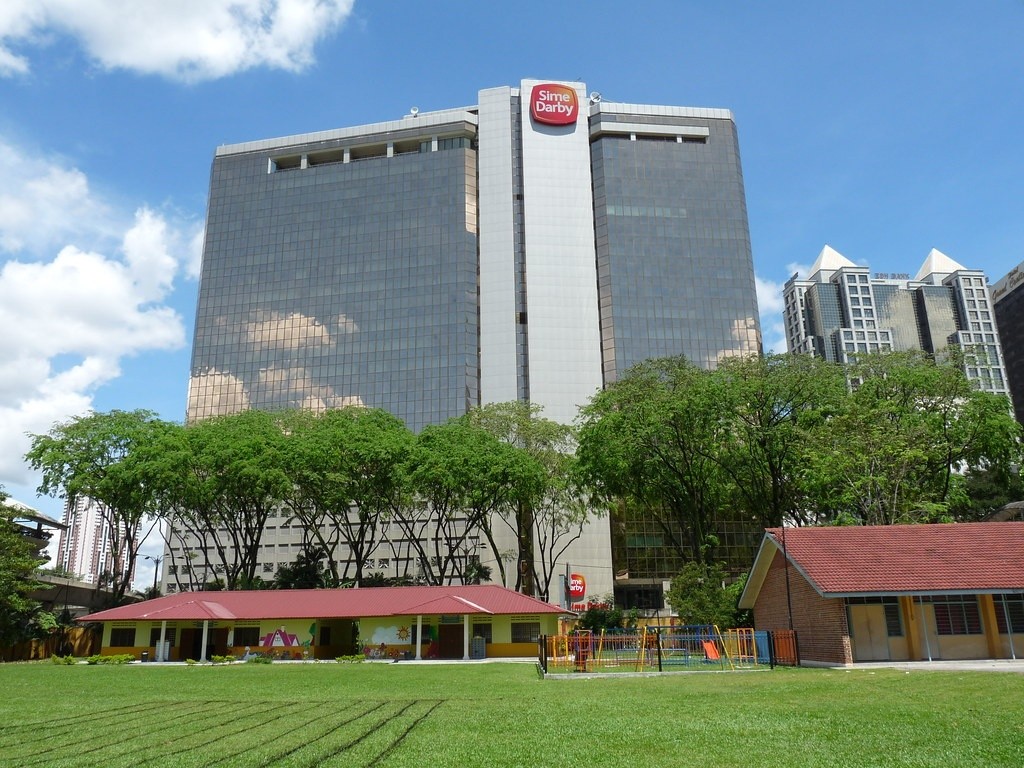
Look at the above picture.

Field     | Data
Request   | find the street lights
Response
[444,542,487,585]
[134,553,184,599]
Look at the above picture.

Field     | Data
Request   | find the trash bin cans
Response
[141,651,149,662]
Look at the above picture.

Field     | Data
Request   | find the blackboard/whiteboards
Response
[154,640,170,661]
